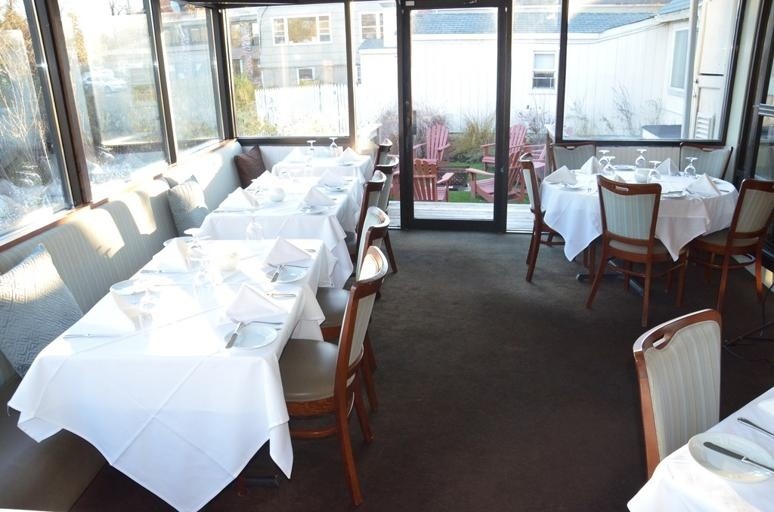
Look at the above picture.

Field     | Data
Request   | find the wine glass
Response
[307,141,316,153]
[329,137,338,150]
[160,228,208,291]
[598,148,698,183]
[109,278,150,335]
[246,214,264,247]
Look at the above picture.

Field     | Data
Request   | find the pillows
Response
[168,175,210,235]
[234,145,265,188]
[1,243,84,378]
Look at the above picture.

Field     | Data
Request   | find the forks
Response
[229,316,282,325]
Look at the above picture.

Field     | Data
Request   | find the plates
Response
[265,268,307,283]
[664,192,686,198]
[304,160,354,214]
[687,430,773,482]
[223,324,277,348]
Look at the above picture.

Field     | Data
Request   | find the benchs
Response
[1,138,244,512]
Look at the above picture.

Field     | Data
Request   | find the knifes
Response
[63,332,121,339]
[701,441,773,473]
[270,264,283,282]
[214,210,241,213]
[224,322,243,349]
[140,269,179,274]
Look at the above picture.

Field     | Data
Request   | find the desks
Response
[654,386,773,511]
[546,166,735,299]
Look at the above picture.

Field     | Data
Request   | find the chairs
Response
[519,152,595,282]
[316,205,390,413]
[632,307,722,478]
[586,173,688,326]
[278,246,389,508]
[345,169,387,300]
[685,177,773,315]
[369,138,400,273]
[547,142,596,247]
[387,126,552,203]
[679,141,734,181]
